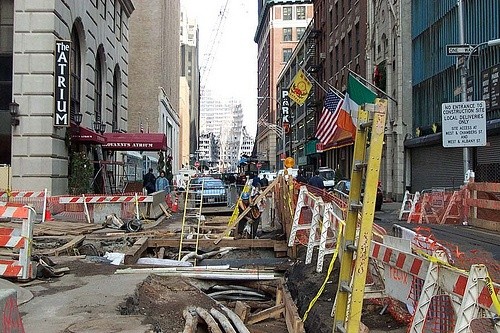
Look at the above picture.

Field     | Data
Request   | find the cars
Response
[330,179,383,211]
[176,167,219,199]
[257,166,299,185]
[193,179,229,207]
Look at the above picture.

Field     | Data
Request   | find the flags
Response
[288,70,313,106]
[315,89,344,147]
[338,76,376,139]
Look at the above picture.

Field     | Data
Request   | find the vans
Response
[317,166,336,191]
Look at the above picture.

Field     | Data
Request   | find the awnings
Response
[102,133,167,151]
[69,122,107,144]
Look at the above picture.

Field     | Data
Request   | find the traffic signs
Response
[455,54,467,70]
[446,44,480,57]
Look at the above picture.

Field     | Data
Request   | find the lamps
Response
[69,109,83,135]
[9,99,19,125]
[93,119,106,136]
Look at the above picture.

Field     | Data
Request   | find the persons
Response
[234,192,260,239]
[229,173,269,188]
[294,170,307,183]
[308,171,324,189]
[142,168,156,194]
[154,171,170,192]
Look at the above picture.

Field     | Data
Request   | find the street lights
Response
[256,96,286,165]
[460,39,500,183]
